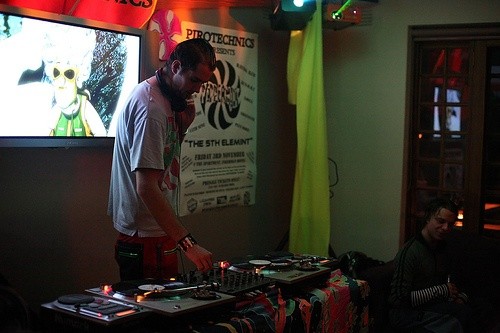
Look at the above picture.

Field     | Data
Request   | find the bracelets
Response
[177,234,198,251]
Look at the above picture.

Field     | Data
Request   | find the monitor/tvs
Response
[0,4,146,148]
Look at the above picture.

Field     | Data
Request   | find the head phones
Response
[155,68,187,112]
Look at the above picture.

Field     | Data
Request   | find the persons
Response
[106,38,216,283]
[387,199,477,333]
[42,41,106,137]
